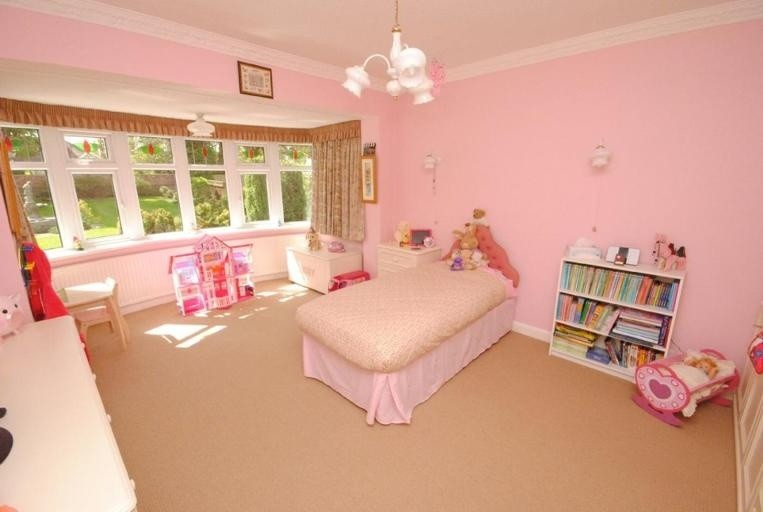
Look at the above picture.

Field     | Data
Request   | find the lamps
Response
[423,153,441,169]
[340,0,435,107]
[185,112,216,137]
[590,138,609,170]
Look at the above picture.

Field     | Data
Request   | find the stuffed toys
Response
[449,221,491,271]
[393,223,433,249]
[0,292,24,336]
[651,239,687,271]
[305,233,323,250]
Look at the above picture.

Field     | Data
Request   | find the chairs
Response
[76,277,130,345]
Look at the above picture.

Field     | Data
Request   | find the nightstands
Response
[377,243,441,279]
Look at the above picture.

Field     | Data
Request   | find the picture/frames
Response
[409,229,432,246]
[237,60,273,100]
[359,155,377,203]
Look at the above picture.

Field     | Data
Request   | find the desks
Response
[1,313,138,512]
[59,283,130,354]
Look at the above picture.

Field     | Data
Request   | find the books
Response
[553,262,680,370]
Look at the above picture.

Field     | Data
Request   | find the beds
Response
[632,349,740,429]
[293,224,521,427]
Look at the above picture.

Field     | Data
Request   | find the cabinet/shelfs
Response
[730,310,762,512]
[547,251,688,383]
[285,241,363,295]
